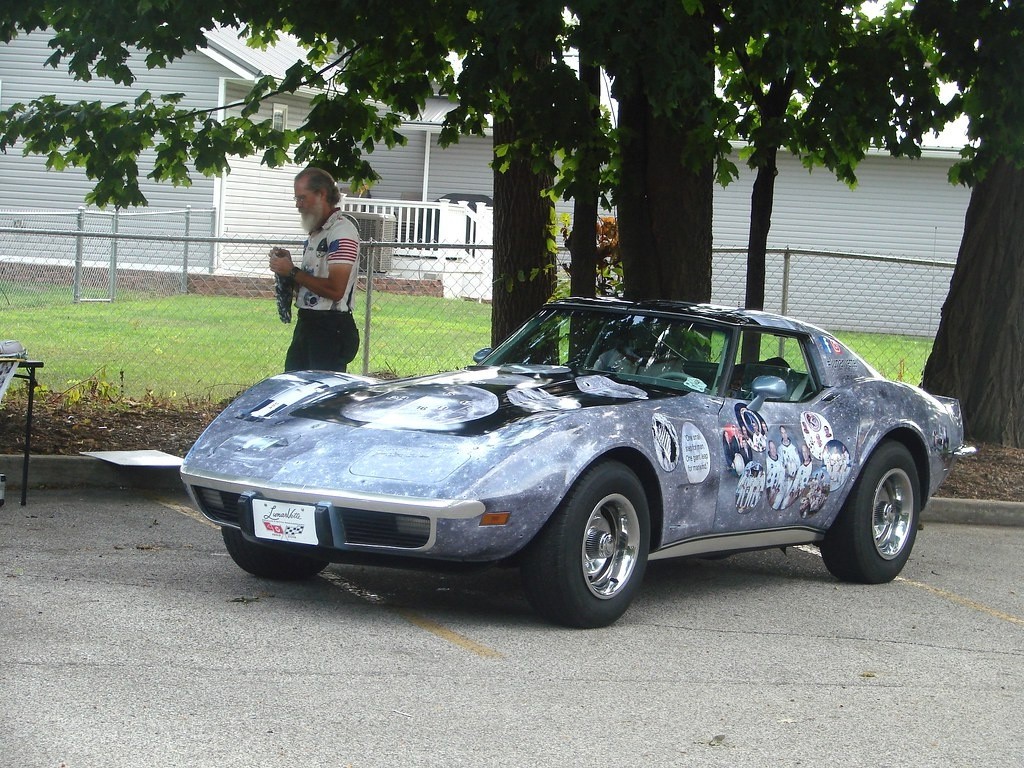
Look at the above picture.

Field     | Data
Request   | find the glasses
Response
[294,190,321,201]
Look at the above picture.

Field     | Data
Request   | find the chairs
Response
[708,363,800,402]
[594,346,684,382]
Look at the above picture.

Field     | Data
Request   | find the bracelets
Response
[290,267,300,278]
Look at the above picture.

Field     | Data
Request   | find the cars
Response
[180,293,977,630]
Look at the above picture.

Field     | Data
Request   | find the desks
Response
[0,358,44,507]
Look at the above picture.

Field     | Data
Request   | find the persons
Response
[269,167,360,374]
[722,415,849,515]
[593,321,685,381]
